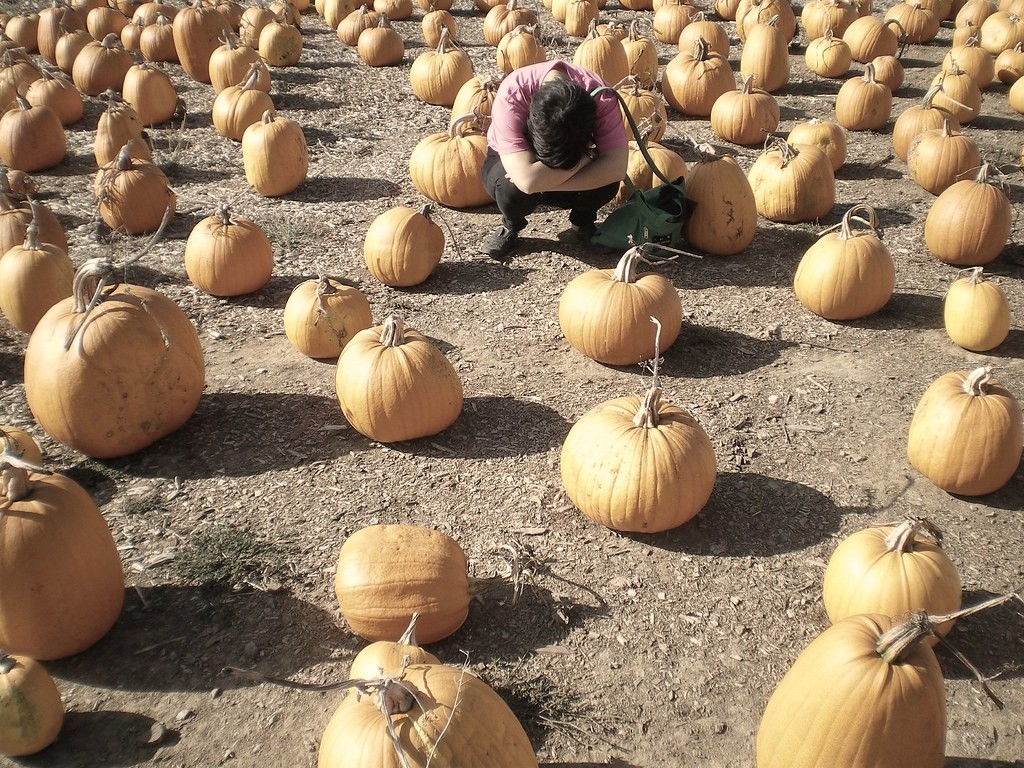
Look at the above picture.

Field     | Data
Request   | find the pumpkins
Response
[0,0,1024,768]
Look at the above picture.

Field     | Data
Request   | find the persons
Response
[480,60,629,259]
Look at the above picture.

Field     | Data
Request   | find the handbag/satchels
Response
[590,176,686,257]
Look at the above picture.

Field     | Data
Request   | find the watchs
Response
[585,150,596,160]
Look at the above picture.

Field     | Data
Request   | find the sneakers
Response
[479,227,518,259]
[572,223,612,255]
